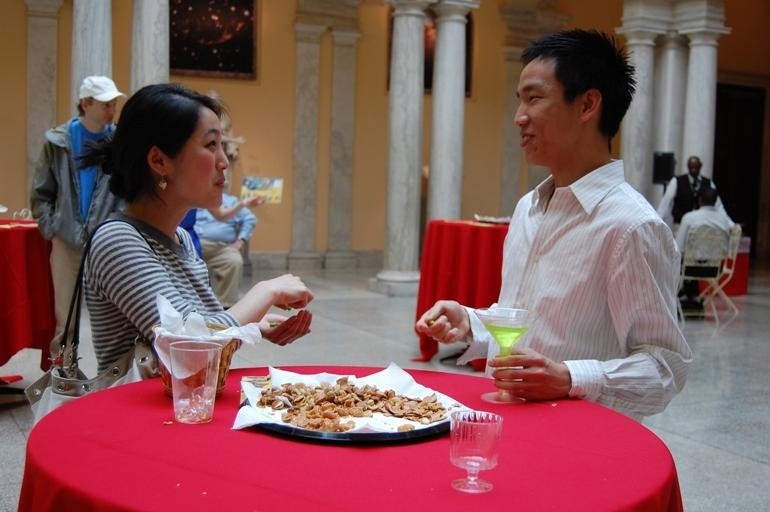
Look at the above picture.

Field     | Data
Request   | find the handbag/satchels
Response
[27,216,158,424]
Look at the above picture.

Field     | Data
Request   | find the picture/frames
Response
[386,7,473,97]
[168,0,258,81]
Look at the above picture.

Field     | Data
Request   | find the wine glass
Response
[474,307,541,403]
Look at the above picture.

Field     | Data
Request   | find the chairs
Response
[696,224,741,313]
[677,223,729,326]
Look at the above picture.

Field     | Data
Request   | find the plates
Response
[238,398,449,442]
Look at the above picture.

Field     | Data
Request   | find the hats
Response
[76,75,126,103]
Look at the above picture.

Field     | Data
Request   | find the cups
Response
[170,342,222,425]
[449,412,502,492]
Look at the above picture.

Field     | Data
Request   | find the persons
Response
[30,76,129,364]
[83,84,314,379]
[414,29,694,424]
[178,196,267,261]
[193,194,258,309]
[676,188,730,320]
[657,156,734,241]
